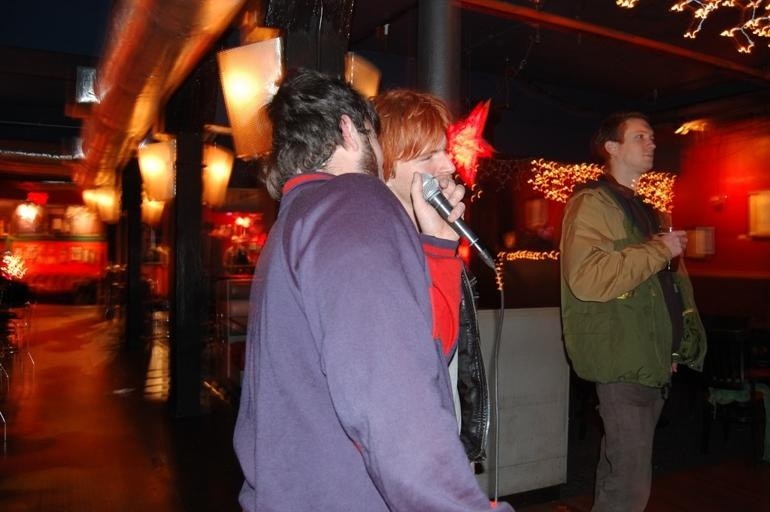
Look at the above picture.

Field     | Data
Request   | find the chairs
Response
[698,328,770,464]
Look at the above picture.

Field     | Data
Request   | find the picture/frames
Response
[746,190,770,239]
[682,226,709,260]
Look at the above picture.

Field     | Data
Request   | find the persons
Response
[233,69,512,511]
[559,110,710,508]
[363,90,488,491]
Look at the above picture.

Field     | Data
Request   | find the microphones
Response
[417,171,497,271]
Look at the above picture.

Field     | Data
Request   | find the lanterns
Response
[214,36,291,163]
[342,53,384,114]
[141,195,167,229]
[202,145,234,211]
[134,137,179,206]
[92,188,124,224]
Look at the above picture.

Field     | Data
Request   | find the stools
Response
[0,277,34,443]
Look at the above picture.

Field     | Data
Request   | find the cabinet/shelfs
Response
[215,276,253,378]
